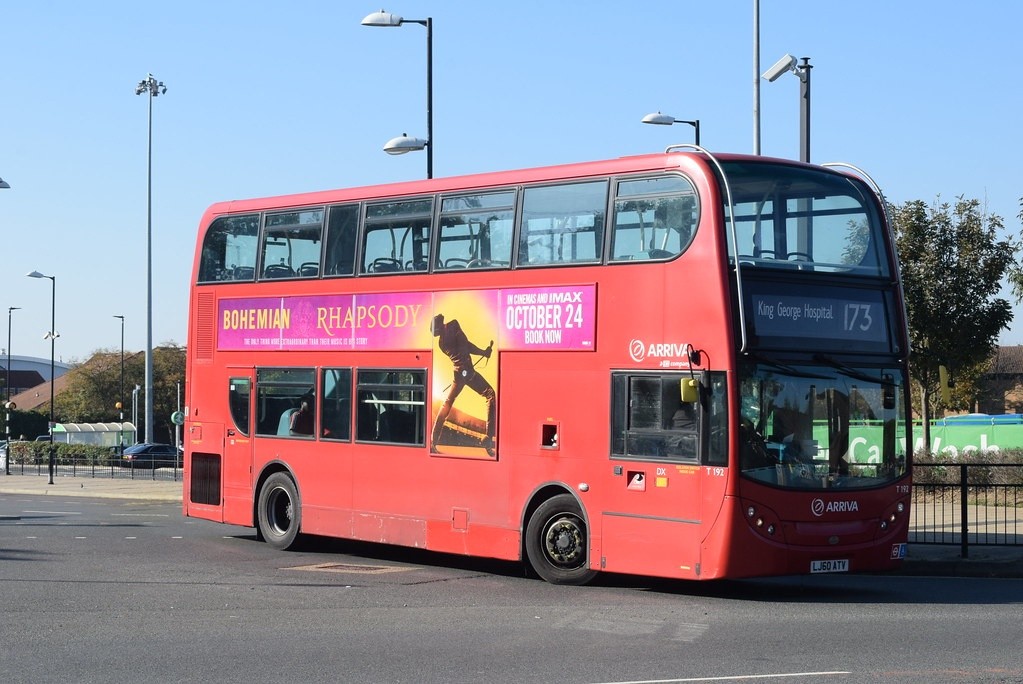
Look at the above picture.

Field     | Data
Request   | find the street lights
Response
[360,8,434,180]
[761,53,816,262]
[26,269,60,485]
[117,315,125,467]
[639,110,701,153]
[132,70,167,443]
[4,306,22,475]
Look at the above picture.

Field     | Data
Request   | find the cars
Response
[114,443,184,468]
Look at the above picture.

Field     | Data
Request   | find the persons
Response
[672,395,763,471]
[430,314,496,458]
[289,393,331,438]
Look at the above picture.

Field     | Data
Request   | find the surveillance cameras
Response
[761,55,797,82]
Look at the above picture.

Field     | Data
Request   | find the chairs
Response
[230,392,425,447]
[208,247,824,281]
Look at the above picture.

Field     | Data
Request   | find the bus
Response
[178,143,921,590]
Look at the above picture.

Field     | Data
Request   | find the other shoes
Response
[481,436,495,457]
[431,444,441,454]
